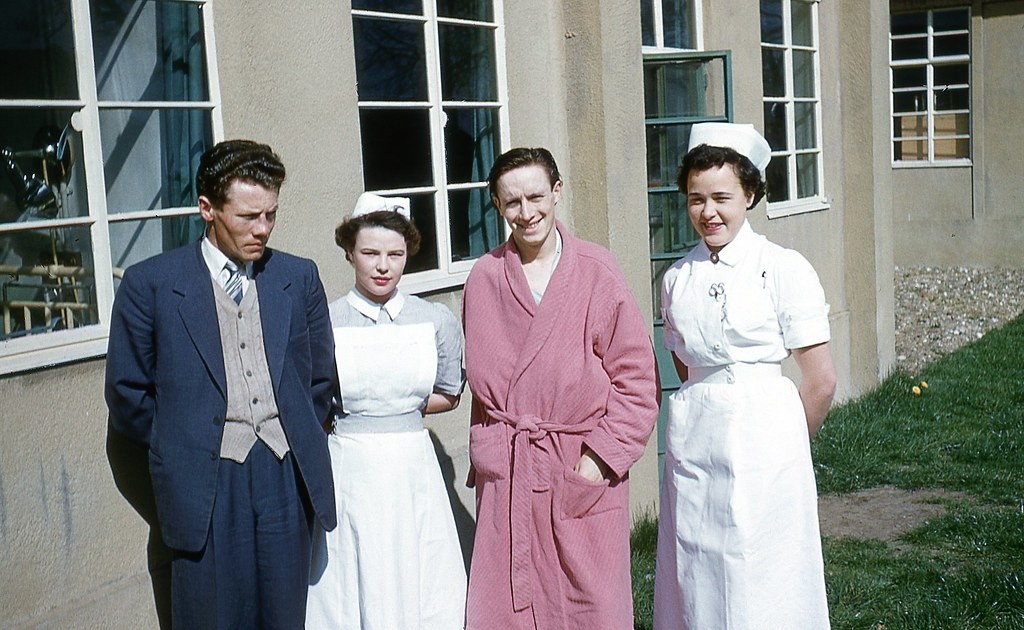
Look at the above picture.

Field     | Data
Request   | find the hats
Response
[351,193,410,221]
[688,123,771,171]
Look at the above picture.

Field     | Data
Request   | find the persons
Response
[104,139,339,630]
[654,123,837,630]
[459,147,661,630]
[304,193,467,630]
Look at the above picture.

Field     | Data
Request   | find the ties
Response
[223,260,243,306]
